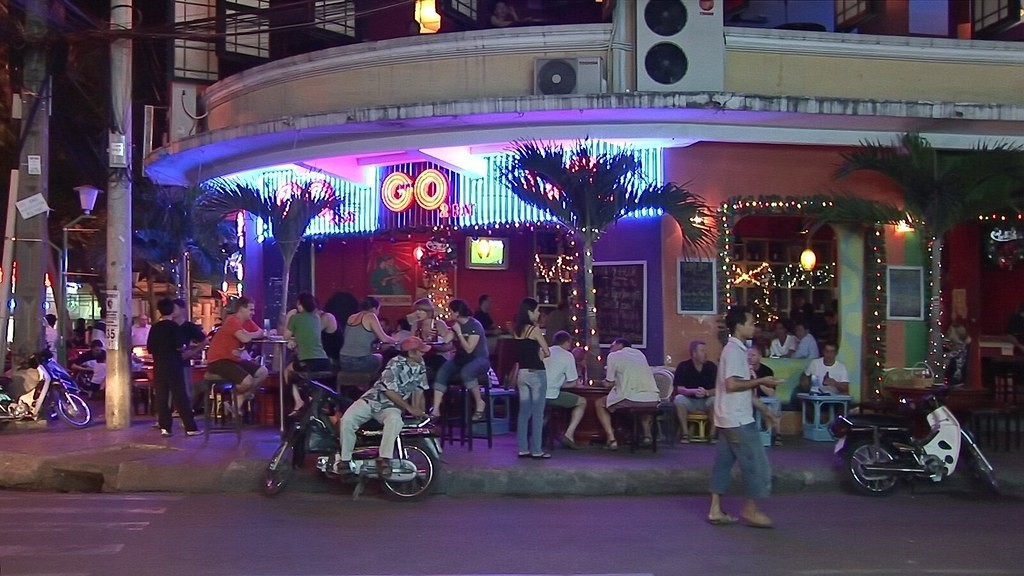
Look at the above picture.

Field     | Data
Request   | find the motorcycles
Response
[261,371,445,502]
[1,344,93,429]
[826,357,998,498]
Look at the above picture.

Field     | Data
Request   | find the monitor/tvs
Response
[464,237,509,271]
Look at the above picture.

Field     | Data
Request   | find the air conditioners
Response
[168,81,196,141]
[636,0,725,92]
[534,57,603,96]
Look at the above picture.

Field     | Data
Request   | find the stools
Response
[204,370,492,453]
[686,410,710,442]
[613,408,665,452]
[541,406,564,449]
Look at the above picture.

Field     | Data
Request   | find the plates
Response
[270,336,283,339]
[291,337,295,339]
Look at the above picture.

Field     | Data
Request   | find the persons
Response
[514,295,552,459]
[397,318,412,333]
[71,339,107,393]
[145,298,209,436]
[427,298,491,422]
[673,340,719,445]
[284,292,344,417]
[594,336,662,451]
[338,337,433,478]
[544,331,588,449]
[473,295,506,336]
[412,298,456,412]
[45,313,60,401]
[376,317,395,343]
[208,317,224,343]
[744,296,839,360]
[337,296,400,393]
[747,344,784,446]
[72,308,107,349]
[801,340,850,424]
[706,308,777,527]
[131,313,152,355]
[207,297,268,419]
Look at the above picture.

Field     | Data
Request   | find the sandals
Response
[680,434,690,443]
[774,434,783,446]
[602,440,620,451]
[639,436,653,448]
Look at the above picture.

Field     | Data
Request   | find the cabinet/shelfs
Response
[527,279,562,328]
[732,238,835,322]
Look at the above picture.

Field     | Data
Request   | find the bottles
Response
[431,323,438,342]
[822,371,829,386]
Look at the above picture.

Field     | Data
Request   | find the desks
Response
[887,387,988,436]
[754,397,781,446]
[560,387,628,447]
[485,335,514,354]
[470,387,515,436]
[374,343,445,350]
[796,393,853,442]
[250,338,295,433]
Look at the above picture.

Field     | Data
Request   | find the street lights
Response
[61,184,103,292]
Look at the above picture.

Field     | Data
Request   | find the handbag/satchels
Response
[507,361,519,389]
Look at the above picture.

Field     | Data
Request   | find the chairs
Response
[495,339,518,383]
[881,362,935,396]
[653,369,674,442]
[967,358,1024,451]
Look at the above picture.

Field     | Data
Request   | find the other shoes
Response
[429,413,442,425]
[172,410,180,417]
[287,401,305,421]
[186,429,204,436]
[472,403,487,422]
[709,513,737,524]
[561,435,581,450]
[223,401,245,417]
[152,422,161,429]
[339,461,356,475]
[375,459,394,478]
[741,511,774,528]
[160,429,174,437]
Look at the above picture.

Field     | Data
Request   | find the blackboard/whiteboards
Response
[591,260,648,348]
[886,265,926,321]
[676,257,717,315]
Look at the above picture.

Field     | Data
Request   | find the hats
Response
[400,336,431,352]
[138,314,147,319]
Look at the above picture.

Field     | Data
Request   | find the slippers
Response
[518,452,552,458]
